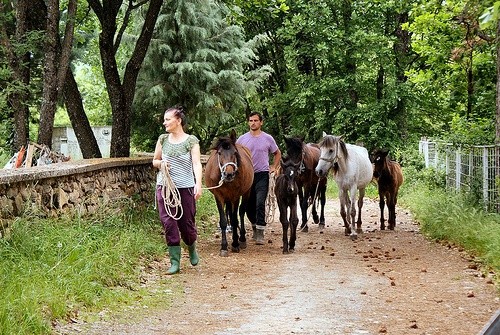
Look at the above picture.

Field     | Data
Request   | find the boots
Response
[187,240,199,266]
[251,224,256,240]
[164,246,181,274]
[255,225,265,245]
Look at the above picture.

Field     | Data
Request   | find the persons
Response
[235,112,281,246]
[152,104,202,275]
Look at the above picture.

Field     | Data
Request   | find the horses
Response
[274,160,301,254]
[315,131,373,238]
[280,134,327,232]
[373,149,403,230]
[205,128,254,257]
